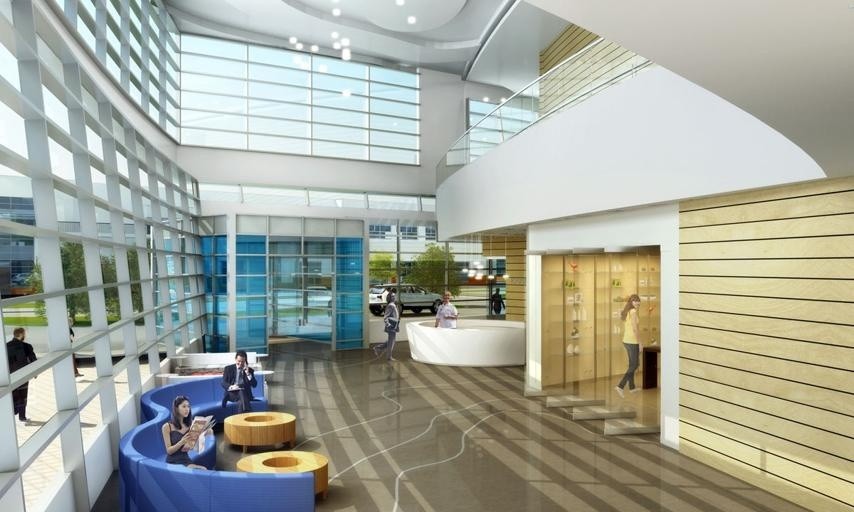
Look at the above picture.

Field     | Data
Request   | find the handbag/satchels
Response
[387,317,399,330]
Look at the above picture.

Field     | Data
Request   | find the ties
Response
[236,367,244,386]
